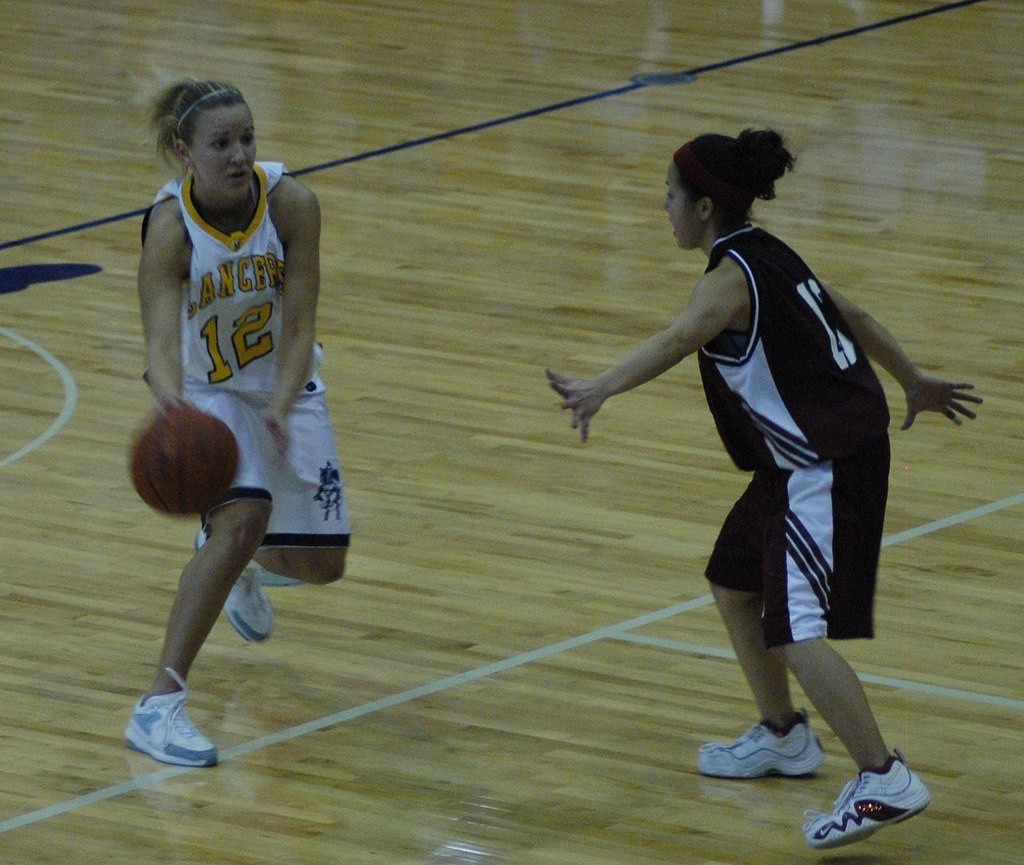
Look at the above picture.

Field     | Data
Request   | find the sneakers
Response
[124,667,217,766]
[194,529,272,642]
[696,706,826,778]
[803,747,932,850]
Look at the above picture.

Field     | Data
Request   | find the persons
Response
[545,128,982,851]
[127,67,354,769]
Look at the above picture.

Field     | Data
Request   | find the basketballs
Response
[126,407,239,516]
